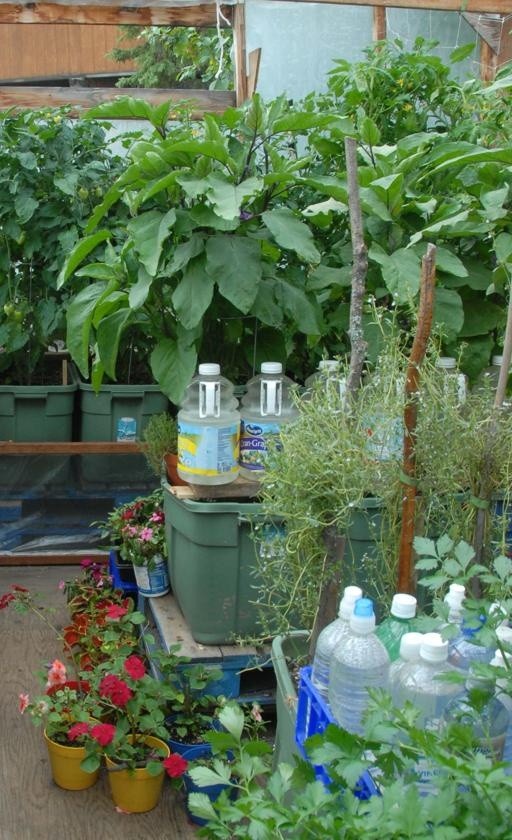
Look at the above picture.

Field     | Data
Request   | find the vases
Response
[130,552,170,598]
[45,718,103,790]
[45,680,92,702]
[105,734,170,813]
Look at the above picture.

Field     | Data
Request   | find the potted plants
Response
[159,649,226,755]
[177,749,236,827]
[139,411,188,486]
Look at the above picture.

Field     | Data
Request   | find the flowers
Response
[19,667,92,739]
[1,585,89,702]
[92,658,180,776]
[163,751,201,777]
[118,499,164,559]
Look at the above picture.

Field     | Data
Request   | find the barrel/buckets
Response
[242,360,306,481]
[470,353,508,415]
[432,357,469,422]
[176,364,243,486]
[361,354,408,461]
[307,359,347,437]
[314,584,512,807]
